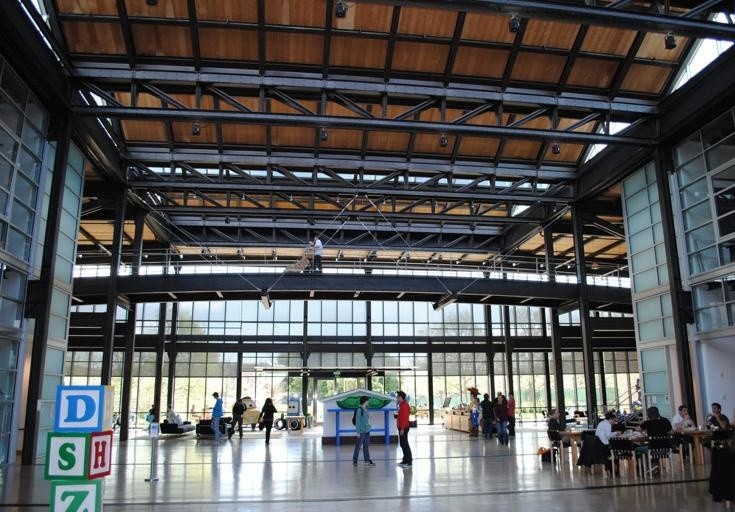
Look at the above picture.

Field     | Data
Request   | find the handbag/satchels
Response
[542,449,558,462]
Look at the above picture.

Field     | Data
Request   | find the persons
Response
[313,235,323,273]
[191,404,201,422]
[583,403,730,476]
[227,398,247,439]
[210,392,224,442]
[394,391,413,468]
[353,396,376,466]
[470,391,516,446]
[171,247,182,274]
[304,241,314,273]
[257,398,277,445]
[547,409,581,449]
[161,406,176,418]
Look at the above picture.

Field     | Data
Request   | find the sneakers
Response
[646,462,659,476]
[353,461,412,468]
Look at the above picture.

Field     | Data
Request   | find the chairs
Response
[535,428,734,512]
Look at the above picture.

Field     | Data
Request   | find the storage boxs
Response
[53,384,115,433]
[43,432,112,481]
[48,480,104,512]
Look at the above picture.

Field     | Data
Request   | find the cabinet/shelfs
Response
[443,409,473,433]
[285,416,305,433]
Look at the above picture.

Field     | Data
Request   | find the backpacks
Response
[352,407,363,425]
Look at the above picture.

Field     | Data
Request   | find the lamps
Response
[439,133,448,146]
[433,291,457,311]
[391,220,397,228]
[591,262,600,269]
[552,143,560,154]
[469,221,478,232]
[320,128,328,140]
[261,287,272,309]
[225,216,230,223]
[664,31,677,49]
[192,120,200,135]
[311,218,315,225]
[335,0,349,18]
[508,13,521,33]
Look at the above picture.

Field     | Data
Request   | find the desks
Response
[327,408,398,446]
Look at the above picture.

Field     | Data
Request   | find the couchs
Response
[160,420,195,434]
[196,420,227,437]
[211,417,234,439]
